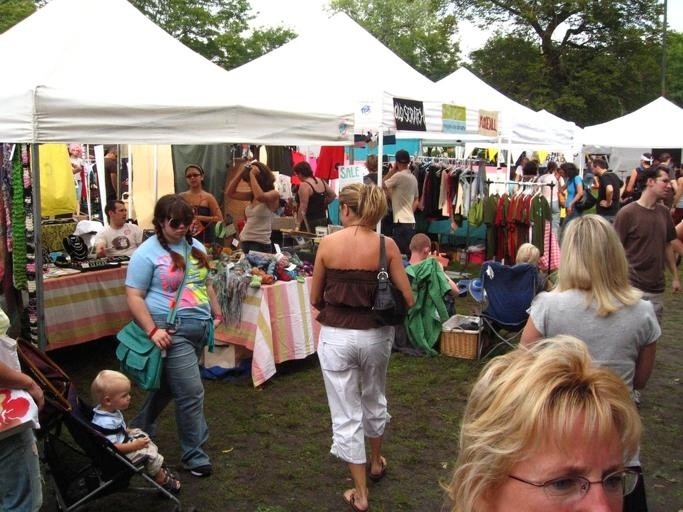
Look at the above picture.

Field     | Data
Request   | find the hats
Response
[396,150,411,163]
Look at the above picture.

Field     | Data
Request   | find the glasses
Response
[243,163,253,170]
[186,173,203,178]
[165,215,193,228]
[507,468,641,502]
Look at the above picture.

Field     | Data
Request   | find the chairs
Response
[479,261,537,360]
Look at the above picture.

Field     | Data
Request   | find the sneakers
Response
[189,465,212,477]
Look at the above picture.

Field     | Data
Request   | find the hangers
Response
[388,156,478,177]
[483,180,547,206]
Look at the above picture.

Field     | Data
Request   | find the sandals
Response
[157,468,181,493]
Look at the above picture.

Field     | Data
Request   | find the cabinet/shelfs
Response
[43,258,133,352]
[212,259,322,388]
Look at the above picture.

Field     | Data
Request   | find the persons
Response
[672,162,683,234]
[364,154,394,238]
[612,168,681,409]
[521,160,538,192]
[440,335,644,511]
[381,151,418,259]
[556,172,567,206]
[88,369,182,497]
[516,214,662,512]
[295,161,337,233]
[70,143,85,214]
[0,302,46,510]
[309,184,417,512]
[96,200,145,260]
[488,242,555,338]
[124,194,223,476]
[533,161,559,237]
[627,152,656,197]
[178,165,223,245]
[590,159,620,222]
[225,158,280,254]
[401,234,461,318]
[515,155,529,182]
[558,162,588,247]
[103,147,120,206]
[654,152,682,217]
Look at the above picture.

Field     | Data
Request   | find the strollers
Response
[16,336,182,512]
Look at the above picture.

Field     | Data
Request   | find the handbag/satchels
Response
[375,235,405,326]
[0,335,40,439]
[467,160,485,226]
[572,176,597,210]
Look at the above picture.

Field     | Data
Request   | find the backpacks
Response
[115,241,191,393]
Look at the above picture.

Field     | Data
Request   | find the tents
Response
[435,66,585,265]
[0,1,355,355]
[229,9,500,238]
[584,96,683,148]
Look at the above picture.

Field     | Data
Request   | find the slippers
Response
[344,488,368,511]
[371,456,387,480]
